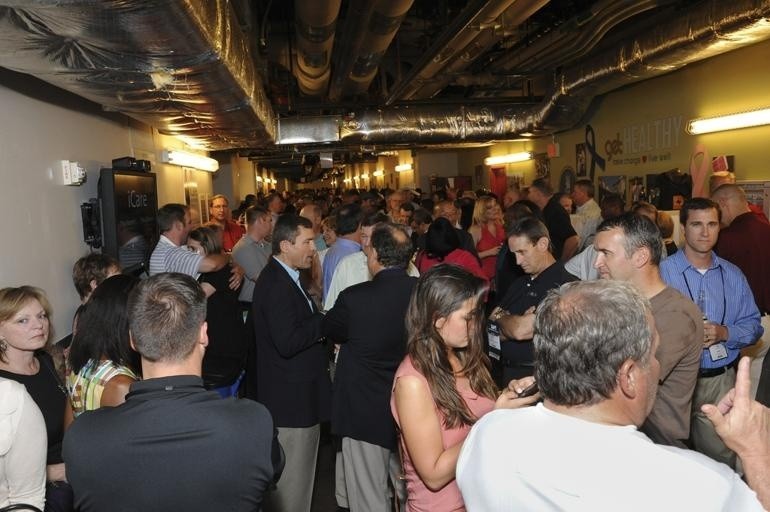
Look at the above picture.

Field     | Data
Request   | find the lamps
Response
[393,163,414,173]
[361,173,370,180]
[684,106,770,135]
[483,150,534,167]
[157,149,220,174]
[373,170,385,177]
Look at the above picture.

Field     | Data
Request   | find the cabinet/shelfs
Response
[430,174,472,196]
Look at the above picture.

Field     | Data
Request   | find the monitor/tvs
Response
[97,168,160,268]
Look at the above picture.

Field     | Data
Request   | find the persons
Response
[1,172,769,512]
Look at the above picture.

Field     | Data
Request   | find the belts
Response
[697,353,741,377]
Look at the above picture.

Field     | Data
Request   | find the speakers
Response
[112,157,136,171]
[137,160,151,172]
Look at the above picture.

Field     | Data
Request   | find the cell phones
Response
[518,382,537,398]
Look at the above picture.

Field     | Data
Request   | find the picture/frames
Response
[558,166,578,197]
